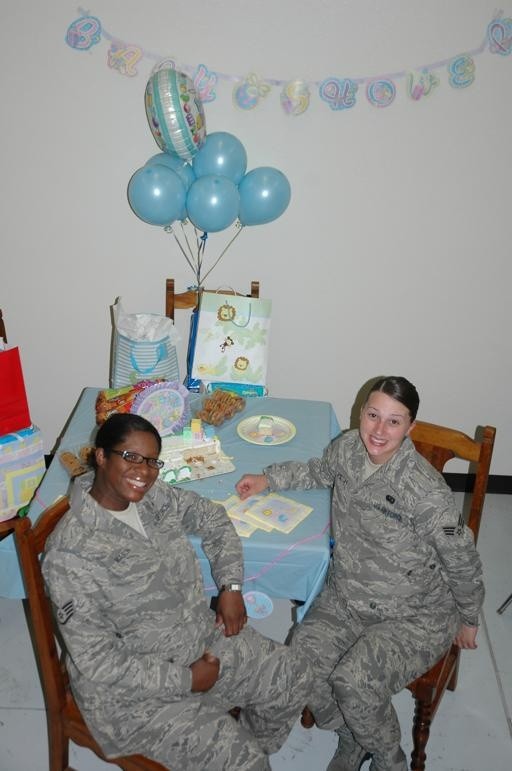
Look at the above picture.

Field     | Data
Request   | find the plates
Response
[237,414,296,446]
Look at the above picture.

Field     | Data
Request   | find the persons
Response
[235,376,487,771]
[38,412,315,771]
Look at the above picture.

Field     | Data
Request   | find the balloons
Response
[187,176,240,235]
[238,167,290,227]
[144,153,196,190]
[144,67,205,161]
[126,168,187,227]
[192,131,248,184]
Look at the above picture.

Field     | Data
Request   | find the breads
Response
[60,447,96,479]
[195,391,244,427]
[93,381,156,425]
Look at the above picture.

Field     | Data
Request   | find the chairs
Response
[302,421,497,770]
[13,495,255,770]
[165,278,260,394]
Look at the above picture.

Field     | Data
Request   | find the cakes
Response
[258,417,274,436]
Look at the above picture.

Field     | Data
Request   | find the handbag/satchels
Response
[0,346,33,436]
[182,285,272,399]
[112,313,179,390]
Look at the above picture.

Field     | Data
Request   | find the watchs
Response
[223,583,242,593]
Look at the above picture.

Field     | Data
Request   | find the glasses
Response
[104,447,165,470]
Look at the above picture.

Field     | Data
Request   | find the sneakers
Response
[326,724,369,771]
